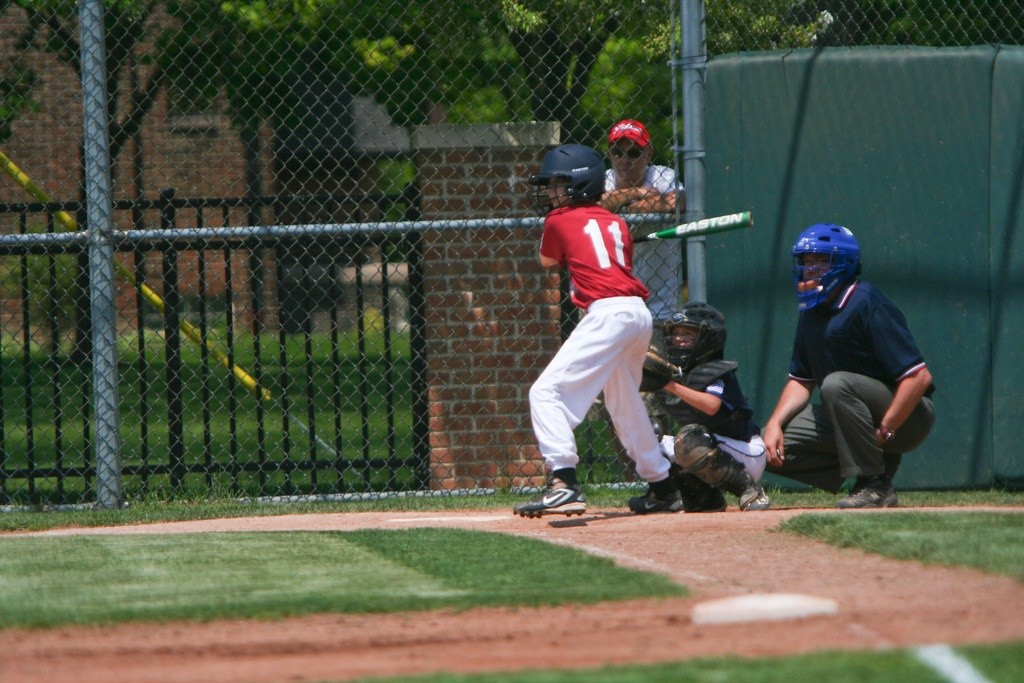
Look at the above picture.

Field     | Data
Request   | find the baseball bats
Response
[633,210,755,243]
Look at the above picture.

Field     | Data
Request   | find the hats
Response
[609,119,651,147]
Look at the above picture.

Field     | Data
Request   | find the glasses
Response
[611,147,645,159]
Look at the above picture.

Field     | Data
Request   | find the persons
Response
[758,221,937,510]
[602,120,688,482]
[511,144,683,519]
[636,298,771,512]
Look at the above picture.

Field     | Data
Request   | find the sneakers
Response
[745,490,770,511]
[836,488,881,508]
[686,495,728,513]
[512,486,586,518]
[882,494,897,507]
[629,495,681,512]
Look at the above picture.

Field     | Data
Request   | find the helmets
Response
[527,143,605,217]
[663,301,726,371]
[791,223,860,311]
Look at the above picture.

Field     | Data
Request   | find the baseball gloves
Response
[639,343,684,393]
